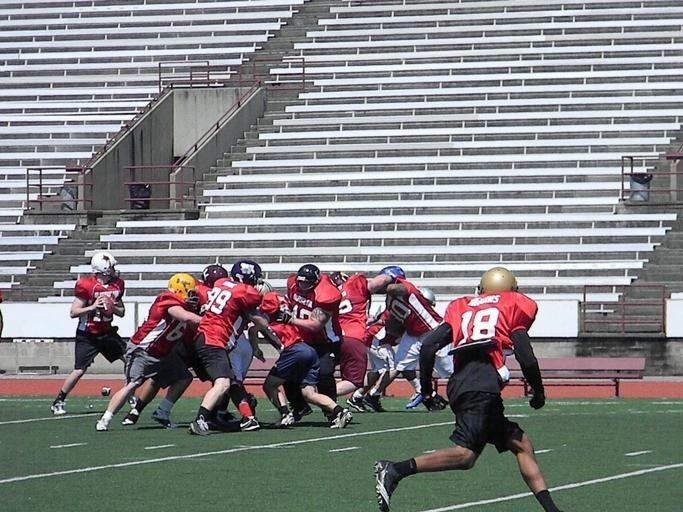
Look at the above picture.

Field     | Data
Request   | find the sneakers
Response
[190,414,210,435]
[347,394,369,413]
[233,416,260,430]
[406,392,424,409]
[51,399,68,416]
[372,460,403,512]
[151,406,175,430]
[336,409,353,429]
[217,410,237,422]
[247,393,258,416]
[128,396,138,408]
[280,412,295,428]
[330,418,337,429]
[362,393,386,412]
[120,408,139,425]
[293,404,313,421]
[95,416,112,431]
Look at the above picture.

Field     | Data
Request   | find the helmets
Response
[253,279,273,296]
[91,251,120,279]
[477,267,518,295]
[168,272,199,300]
[295,264,321,295]
[330,272,350,288]
[201,263,228,288]
[418,287,436,307]
[231,258,262,285]
[378,266,406,285]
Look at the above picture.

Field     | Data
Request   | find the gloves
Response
[276,309,293,325]
[529,392,545,410]
[420,389,449,411]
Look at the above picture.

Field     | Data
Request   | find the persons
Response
[227,328,262,431]
[345,287,436,414]
[277,264,342,424]
[48,251,139,416]
[374,266,510,410]
[372,266,560,512]
[190,259,264,435]
[95,273,203,431]
[253,280,354,429]
[120,264,229,425]
[330,270,394,397]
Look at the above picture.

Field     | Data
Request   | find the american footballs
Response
[97,294,112,318]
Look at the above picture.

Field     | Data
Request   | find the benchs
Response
[192,357,646,398]
